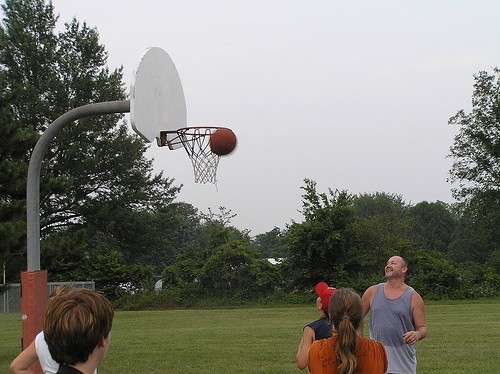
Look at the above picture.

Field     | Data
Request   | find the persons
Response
[43,288,114,374]
[356,255,427,374]
[293,282,337,370]
[308,288,389,374]
[9,282,97,373]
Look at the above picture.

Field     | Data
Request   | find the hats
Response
[315,282,337,314]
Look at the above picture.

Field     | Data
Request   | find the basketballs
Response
[209,128,237,156]
